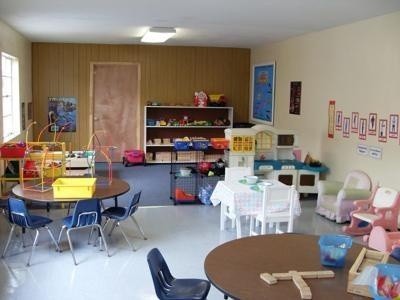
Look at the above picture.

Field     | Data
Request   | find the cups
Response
[257,181,274,191]
[244,175,259,184]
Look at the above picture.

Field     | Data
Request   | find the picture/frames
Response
[250,60,277,127]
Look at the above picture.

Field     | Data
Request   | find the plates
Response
[250,185,259,191]
[240,179,260,186]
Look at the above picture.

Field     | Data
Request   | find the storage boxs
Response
[193,138,209,151]
[155,151,177,163]
[211,138,230,149]
[174,137,190,151]
[206,154,224,162]
[177,150,205,163]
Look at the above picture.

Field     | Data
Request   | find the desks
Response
[11,176,131,251]
[203,232,400,300]
[218,177,298,239]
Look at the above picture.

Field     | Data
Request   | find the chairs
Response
[55,197,111,266]
[314,169,373,225]
[146,248,212,300]
[0,195,64,269]
[248,184,297,238]
[341,180,399,241]
[0,190,13,231]
[100,191,150,255]
[224,166,256,230]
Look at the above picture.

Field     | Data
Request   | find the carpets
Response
[0,161,201,209]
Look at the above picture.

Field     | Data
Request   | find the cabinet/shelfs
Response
[258,160,319,198]
[0,152,67,195]
[143,105,234,167]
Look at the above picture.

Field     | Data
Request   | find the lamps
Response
[140,26,178,46]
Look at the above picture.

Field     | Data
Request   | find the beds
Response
[29,150,97,177]
